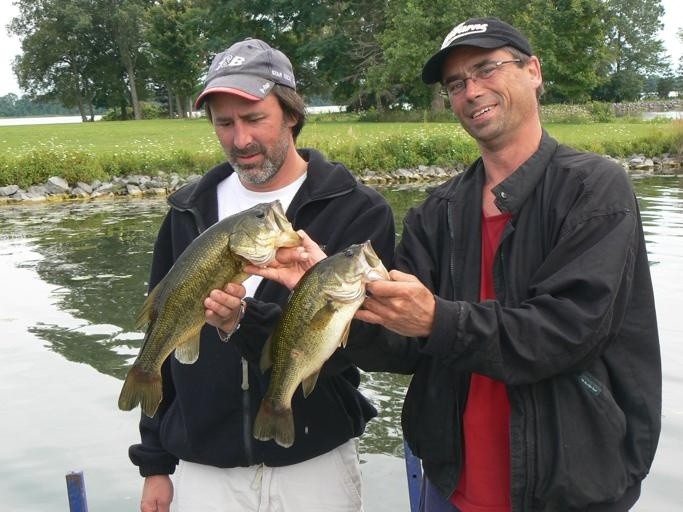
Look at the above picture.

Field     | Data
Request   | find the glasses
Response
[437,59,523,99]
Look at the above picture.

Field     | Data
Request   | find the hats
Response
[191,35,298,111]
[420,16,535,86]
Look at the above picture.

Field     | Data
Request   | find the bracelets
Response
[214,301,244,343]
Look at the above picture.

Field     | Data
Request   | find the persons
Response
[242,15,663,512]
[129,36,396,512]
